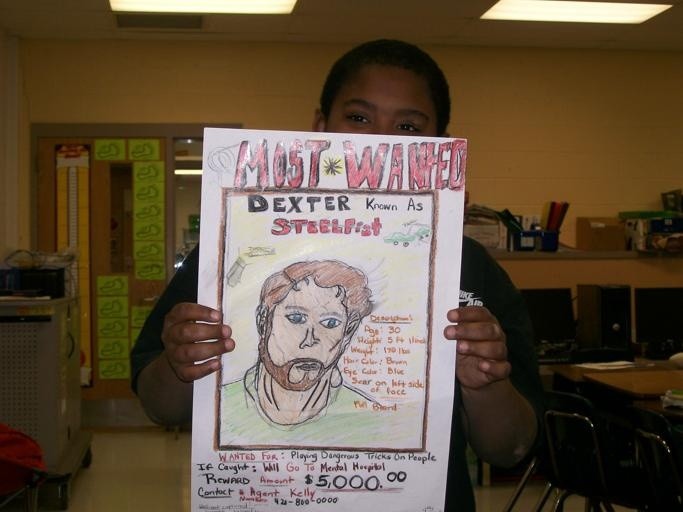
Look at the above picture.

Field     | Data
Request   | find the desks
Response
[529,357,683,425]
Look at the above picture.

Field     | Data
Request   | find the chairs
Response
[545,410,633,512]
[623,406,683,512]
[505,389,630,512]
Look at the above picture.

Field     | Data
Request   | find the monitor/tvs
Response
[520,288,576,359]
[635,287,683,360]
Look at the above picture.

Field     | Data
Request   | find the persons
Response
[220,260,382,447]
[131,39,539,512]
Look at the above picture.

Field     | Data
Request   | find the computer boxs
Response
[577,284,631,363]
